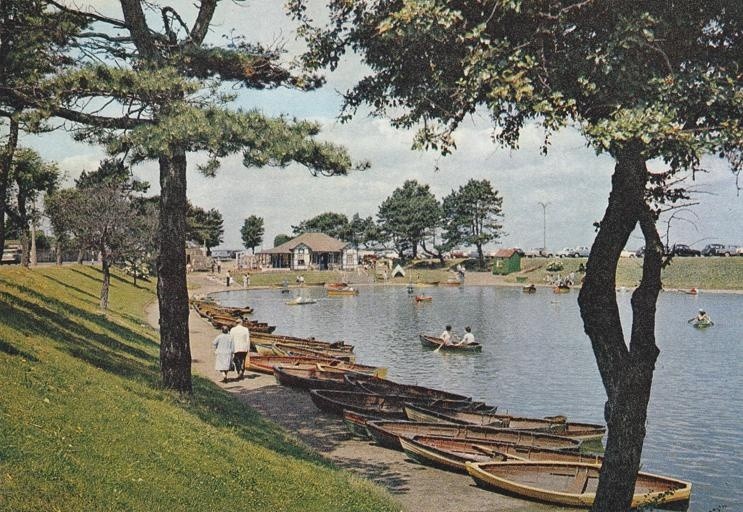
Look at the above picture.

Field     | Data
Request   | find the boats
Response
[682,288,715,330]
[554,286,571,294]
[522,287,536,293]
[327,290,360,296]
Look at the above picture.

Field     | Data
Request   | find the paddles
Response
[433,342,444,352]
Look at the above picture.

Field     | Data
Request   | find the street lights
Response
[537,199,553,250]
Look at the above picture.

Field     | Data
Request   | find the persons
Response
[456,327,476,346]
[226,270,232,287]
[441,325,454,345]
[697,309,709,324]
[244,272,250,288]
[544,269,575,287]
[213,318,250,381]
[457,264,465,277]
[297,275,304,285]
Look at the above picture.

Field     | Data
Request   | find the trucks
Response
[1,244,24,264]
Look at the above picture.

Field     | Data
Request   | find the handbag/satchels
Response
[243,353,250,369]
[228,365,235,371]
